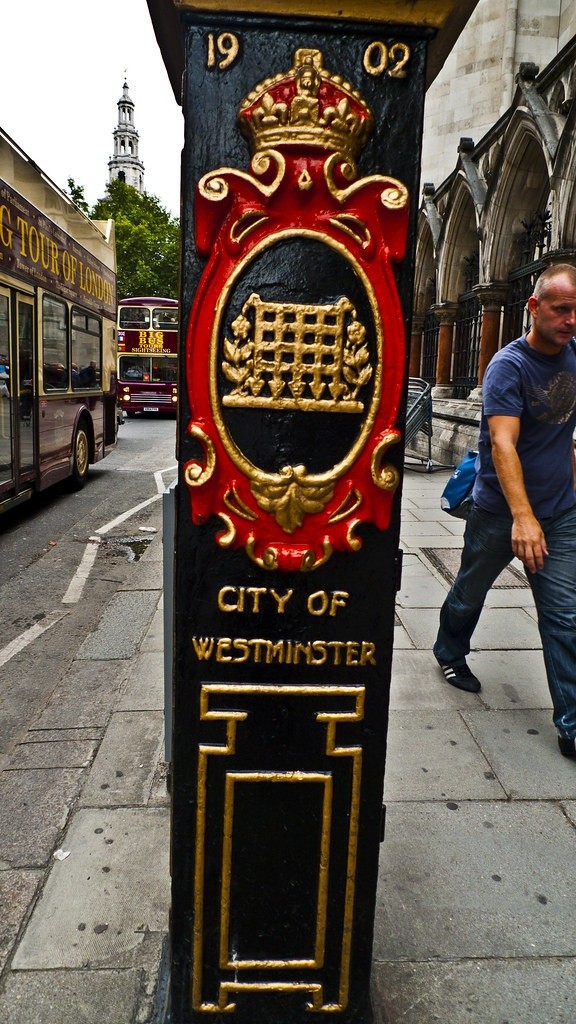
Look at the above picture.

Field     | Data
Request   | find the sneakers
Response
[437,660,481,693]
[558,734,576,759]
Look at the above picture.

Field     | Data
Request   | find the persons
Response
[431,263,575,765]
[59,360,102,388]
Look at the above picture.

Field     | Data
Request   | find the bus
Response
[117,297,178,417]
[0,128,119,513]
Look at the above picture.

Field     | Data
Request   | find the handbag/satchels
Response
[440,449,481,521]
